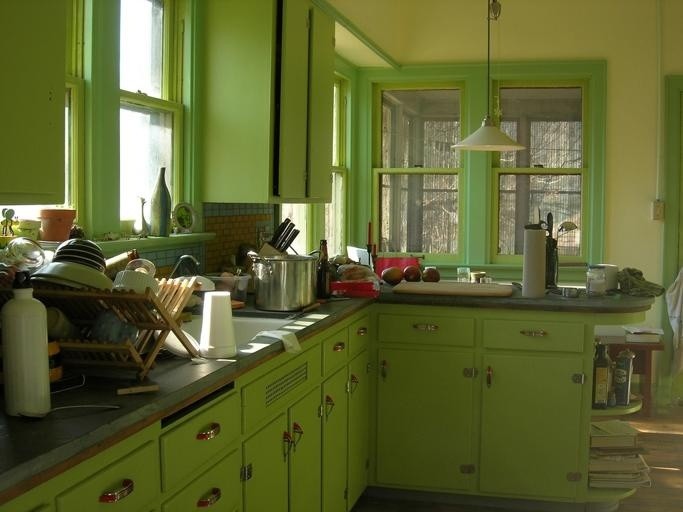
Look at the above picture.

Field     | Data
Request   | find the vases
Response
[16,220,41,242]
[39,209,76,242]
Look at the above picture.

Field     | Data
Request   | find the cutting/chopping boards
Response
[392,280,513,297]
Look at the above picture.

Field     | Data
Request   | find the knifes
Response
[284,302,320,319]
[269,217,291,246]
[280,228,300,253]
[274,222,295,250]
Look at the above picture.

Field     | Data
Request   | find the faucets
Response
[166,254,202,279]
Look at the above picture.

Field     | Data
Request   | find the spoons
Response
[17,402,121,421]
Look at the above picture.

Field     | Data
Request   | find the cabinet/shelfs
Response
[0,0,69,205]
[580,301,650,511]
[158,375,241,512]
[203,0,334,202]
[240,322,323,512]
[0,416,159,512]
[321,298,375,512]
[373,299,589,511]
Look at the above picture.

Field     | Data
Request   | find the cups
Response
[456,267,470,283]
[199,287,239,358]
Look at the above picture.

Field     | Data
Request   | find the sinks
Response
[174,312,299,358]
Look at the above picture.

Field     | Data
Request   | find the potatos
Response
[382,267,403,285]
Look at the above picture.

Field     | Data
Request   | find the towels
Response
[250,328,302,355]
[664,267,682,380]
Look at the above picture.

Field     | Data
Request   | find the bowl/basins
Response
[112,269,158,295]
[52,237,108,273]
[202,272,251,302]
[27,260,116,297]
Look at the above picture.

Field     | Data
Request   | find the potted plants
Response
[523,212,577,291]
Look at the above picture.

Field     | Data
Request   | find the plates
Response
[155,328,199,357]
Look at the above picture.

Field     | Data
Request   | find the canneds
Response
[586,265,607,298]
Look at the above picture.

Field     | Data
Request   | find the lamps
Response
[449,0,527,152]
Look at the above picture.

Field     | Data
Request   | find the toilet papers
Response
[522,227,549,301]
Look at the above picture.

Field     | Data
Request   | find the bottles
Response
[0,270,53,420]
[614,347,636,407]
[317,239,332,300]
[590,343,612,411]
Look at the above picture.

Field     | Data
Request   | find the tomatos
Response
[404,265,422,282]
[423,268,440,282]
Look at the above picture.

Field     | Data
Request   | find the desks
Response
[590,332,665,417]
